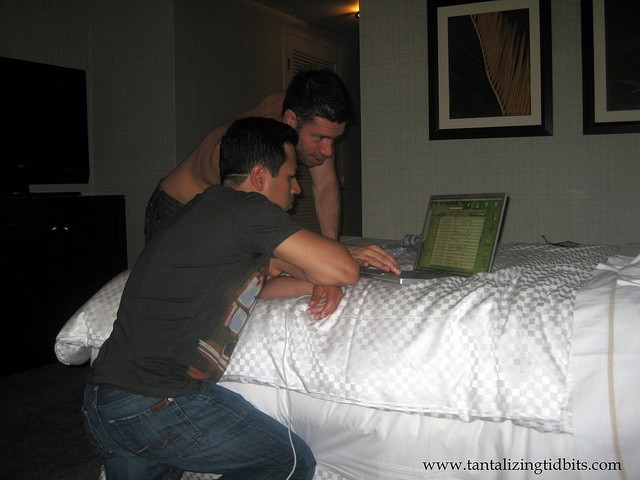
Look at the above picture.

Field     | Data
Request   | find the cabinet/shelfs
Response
[0,196,127,376]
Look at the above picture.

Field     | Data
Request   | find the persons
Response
[143,67,404,277]
[80,114,362,479]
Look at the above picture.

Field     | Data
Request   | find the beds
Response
[55,235,640,480]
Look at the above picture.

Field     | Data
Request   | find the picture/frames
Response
[580,0,640,135]
[427,0,553,140]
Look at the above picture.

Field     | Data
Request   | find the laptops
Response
[360,192,508,286]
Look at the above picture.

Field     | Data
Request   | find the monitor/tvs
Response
[0,56,90,198]
